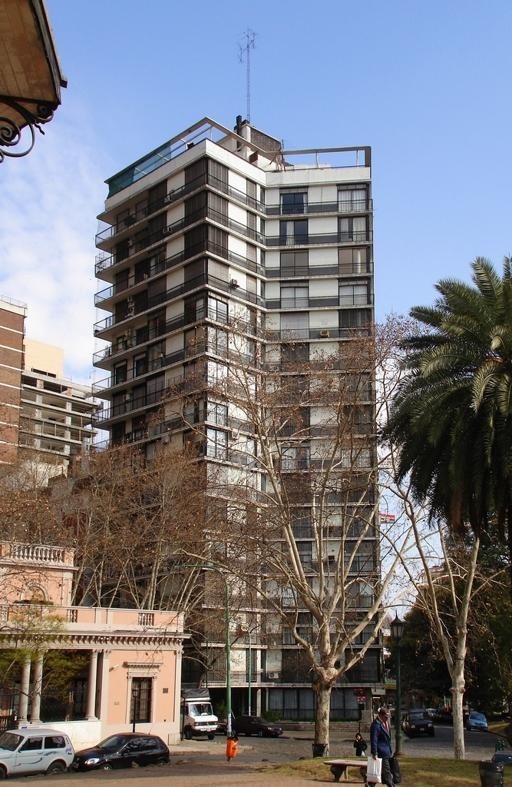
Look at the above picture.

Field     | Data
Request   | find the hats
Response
[379,707,392,718]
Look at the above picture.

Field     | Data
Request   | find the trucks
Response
[181,688,219,740]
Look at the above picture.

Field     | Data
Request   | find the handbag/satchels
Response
[365,756,383,783]
[389,757,401,783]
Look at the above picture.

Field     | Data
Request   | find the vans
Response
[215,707,236,734]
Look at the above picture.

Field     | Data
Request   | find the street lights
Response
[174,564,232,737]
[391,609,405,754]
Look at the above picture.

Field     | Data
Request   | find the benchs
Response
[323,759,369,783]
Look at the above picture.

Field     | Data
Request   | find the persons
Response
[353,732,367,758]
[364,706,395,786]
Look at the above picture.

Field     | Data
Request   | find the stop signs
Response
[361,696,367,702]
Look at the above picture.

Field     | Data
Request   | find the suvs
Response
[0,729,76,778]
[73,733,171,770]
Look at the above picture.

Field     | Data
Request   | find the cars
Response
[466,712,488,732]
[402,708,453,737]
[234,715,283,737]
